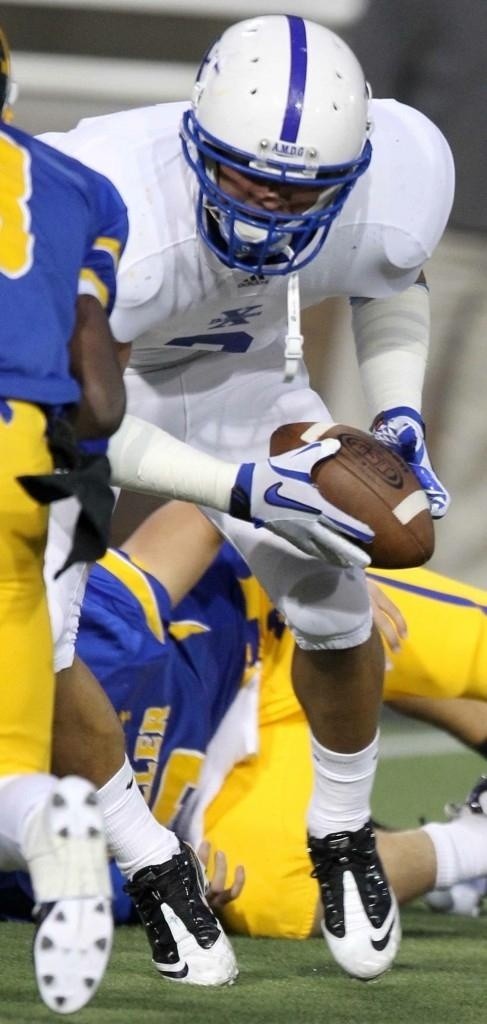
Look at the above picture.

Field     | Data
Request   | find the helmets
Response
[180,14,375,276]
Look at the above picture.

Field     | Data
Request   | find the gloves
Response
[374,406,450,518]
[229,438,375,570]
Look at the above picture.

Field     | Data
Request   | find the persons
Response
[28,14,457,988]
[0,499,486,944]
[0,36,126,1012]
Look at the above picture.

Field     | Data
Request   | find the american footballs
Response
[270,421,433,568]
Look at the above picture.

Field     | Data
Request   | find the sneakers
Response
[122,831,238,987]
[18,774,115,1014]
[307,819,402,980]
